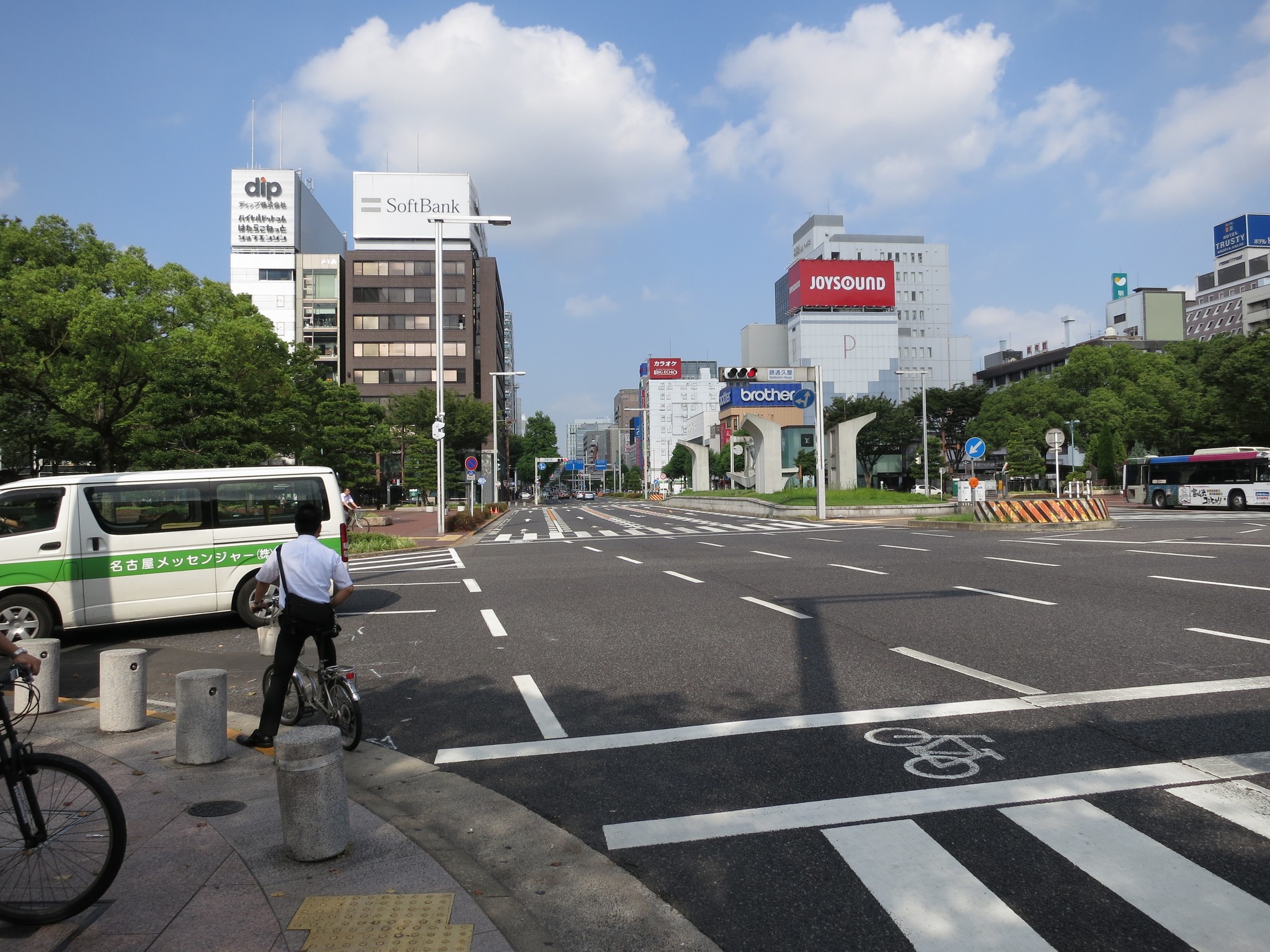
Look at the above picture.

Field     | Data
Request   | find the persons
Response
[711,486,715,491]
[340,487,361,526]
[0,631,41,676]
[277,493,287,508]
[235,503,354,747]
[0,496,54,532]
[515,491,522,501]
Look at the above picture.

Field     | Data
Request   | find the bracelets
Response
[10,647,28,660]
[2,518,7,523]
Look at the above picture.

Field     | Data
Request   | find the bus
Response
[273,483,293,506]
[1122,446,1270,510]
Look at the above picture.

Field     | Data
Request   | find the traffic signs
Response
[565,460,584,470]
[595,460,607,470]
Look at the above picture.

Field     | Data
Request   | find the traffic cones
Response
[493,507,498,513]
[490,507,493,513]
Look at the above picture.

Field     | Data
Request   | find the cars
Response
[910,485,942,495]
[655,488,670,494]
[572,489,596,501]
[600,489,635,494]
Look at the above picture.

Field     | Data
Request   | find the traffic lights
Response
[432,421,446,442]
[577,474,583,477]
[724,367,757,379]
[915,456,921,465]
[557,458,567,466]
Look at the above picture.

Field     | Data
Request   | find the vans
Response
[0,466,347,634]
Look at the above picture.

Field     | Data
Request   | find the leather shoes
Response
[235,729,274,748]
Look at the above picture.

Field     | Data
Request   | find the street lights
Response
[624,406,667,499]
[1064,418,1081,472]
[604,427,635,491]
[425,213,515,533]
[489,371,527,502]
[643,449,656,491]
[656,440,673,495]
[894,370,930,500]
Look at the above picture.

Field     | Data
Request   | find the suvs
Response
[282,493,297,498]
[540,492,553,498]
[558,491,570,499]
[521,492,533,499]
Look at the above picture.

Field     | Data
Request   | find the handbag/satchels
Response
[257,606,305,657]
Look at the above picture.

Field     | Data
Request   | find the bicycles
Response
[346,507,370,536]
[641,491,656,494]
[0,662,133,927]
[252,596,364,751]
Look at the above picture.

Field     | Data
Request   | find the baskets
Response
[356,510,366,517]
